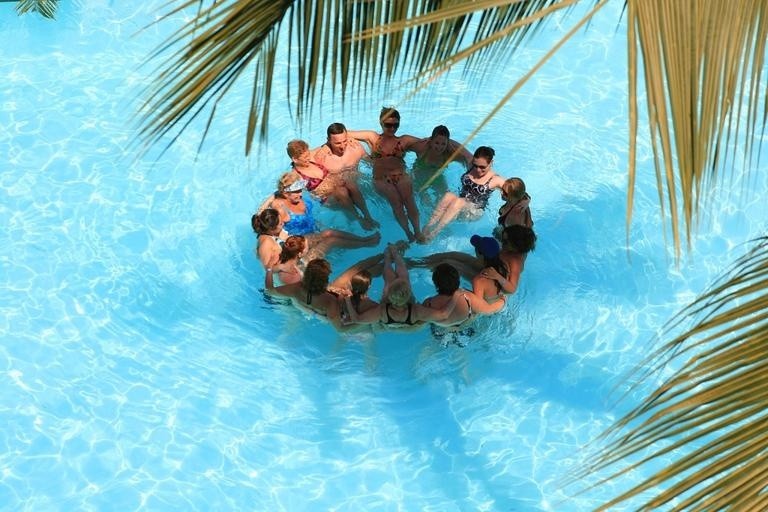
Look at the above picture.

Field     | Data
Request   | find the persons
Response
[251,106,536,345]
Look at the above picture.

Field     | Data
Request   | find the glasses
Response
[502,187,508,197]
[472,161,490,170]
[383,120,399,129]
[501,232,510,246]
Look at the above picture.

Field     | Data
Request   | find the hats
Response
[470,234,500,259]
[283,178,309,192]
